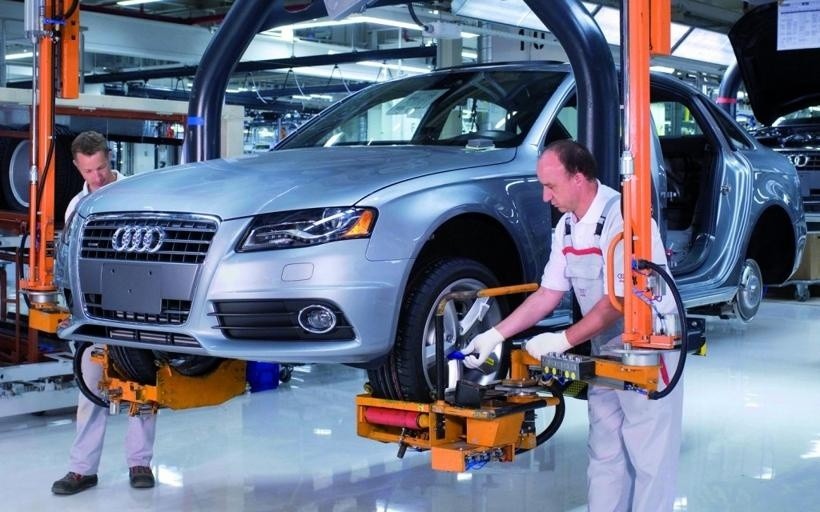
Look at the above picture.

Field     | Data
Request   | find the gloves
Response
[460,325,506,370]
[524,329,573,364]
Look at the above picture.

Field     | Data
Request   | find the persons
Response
[50,129,159,496]
[459,139,688,512]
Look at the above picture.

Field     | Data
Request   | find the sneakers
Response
[128,465,156,489]
[52,471,99,495]
